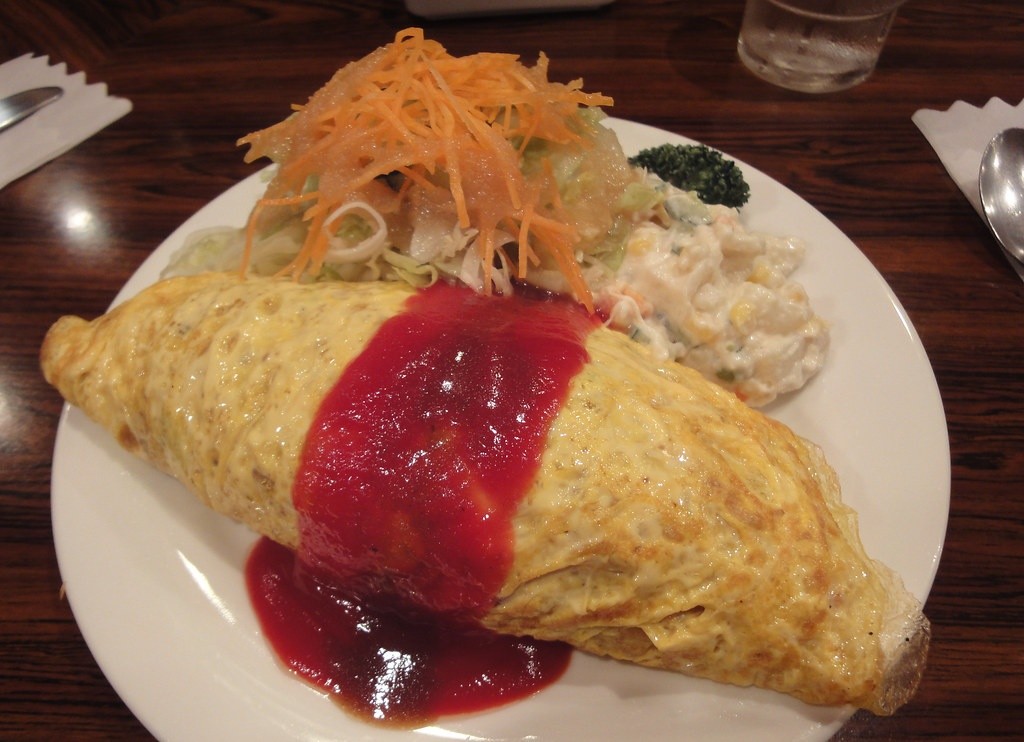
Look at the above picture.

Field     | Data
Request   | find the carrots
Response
[236,26,613,308]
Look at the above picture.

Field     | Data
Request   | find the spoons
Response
[979,127,1024,267]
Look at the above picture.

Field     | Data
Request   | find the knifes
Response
[0,87,64,131]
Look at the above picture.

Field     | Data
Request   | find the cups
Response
[737,0,900,93]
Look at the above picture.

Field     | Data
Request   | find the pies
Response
[38,269,928,715]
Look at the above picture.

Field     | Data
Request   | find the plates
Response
[50,116,951,742]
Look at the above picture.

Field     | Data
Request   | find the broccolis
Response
[630,144,749,210]
